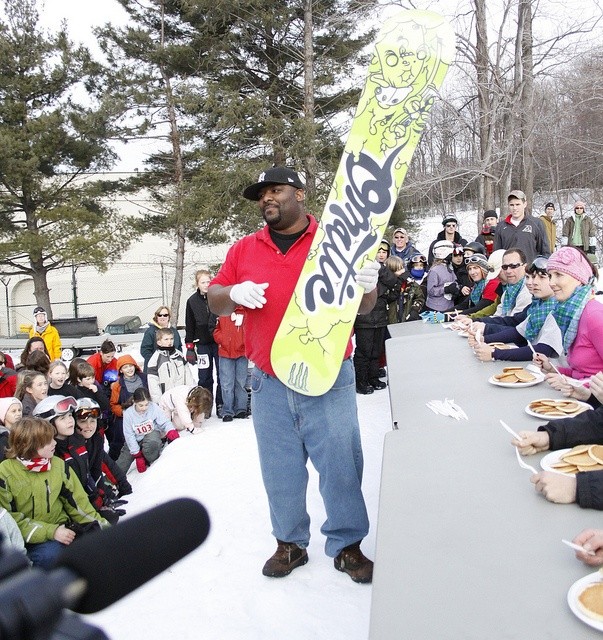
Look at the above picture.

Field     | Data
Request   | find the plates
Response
[487,373,544,388]
[540,448,578,477]
[457,330,469,337]
[473,344,519,355]
[524,398,594,420]
[566,570,602,630]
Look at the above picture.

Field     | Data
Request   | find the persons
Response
[68,359,85,384]
[76,358,110,424]
[144,328,197,402]
[0,395,22,459]
[538,201,557,251]
[561,373,603,401]
[47,362,67,394]
[0,415,113,563]
[453,250,504,330]
[373,240,387,265]
[212,306,247,422]
[110,354,147,415]
[30,395,125,524]
[454,254,487,327]
[409,253,427,284]
[466,264,531,335]
[567,532,602,569]
[0,352,16,397]
[0,503,107,640]
[494,189,551,260]
[426,239,458,313]
[510,406,601,509]
[15,337,48,367]
[184,270,223,420]
[443,242,484,321]
[429,215,467,261]
[208,169,381,582]
[158,386,214,434]
[75,399,131,504]
[457,249,532,333]
[27,307,62,362]
[391,229,417,256]
[139,306,181,373]
[562,201,596,252]
[453,243,465,306]
[384,257,403,323]
[469,256,557,360]
[356,269,387,391]
[121,388,179,472]
[406,255,427,296]
[85,341,116,384]
[475,208,498,256]
[422,243,488,322]
[533,246,602,391]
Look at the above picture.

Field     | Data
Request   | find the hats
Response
[453,242,464,254]
[484,210,496,219]
[73,396,102,431]
[0,396,22,423]
[545,202,555,210]
[31,394,77,436]
[508,189,526,200]
[462,241,484,253]
[32,306,47,322]
[464,253,486,271]
[441,211,456,224]
[486,248,505,279]
[243,168,302,200]
[392,227,406,235]
[574,201,585,212]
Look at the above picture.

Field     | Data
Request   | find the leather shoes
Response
[234,411,248,417]
[379,368,384,376]
[371,378,385,389]
[222,415,232,421]
[356,380,373,393]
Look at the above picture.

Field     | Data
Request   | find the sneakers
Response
[334,544,373,582]
[262,541,307,576]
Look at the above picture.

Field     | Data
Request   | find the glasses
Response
[464,256,487,264]
[74,407,99,418]
[394,236,404,239]
[378,243,387,249]
[156,314,168,316]
[575,206,583,208]
[35,395,76,418]
[410,255,426,262]
[501,262,523,269]
[446,224,455,226]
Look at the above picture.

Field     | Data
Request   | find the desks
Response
[385,337,589,429]
[386,320,466,337]
[368,428,601,639]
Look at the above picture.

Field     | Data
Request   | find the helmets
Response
[378,239,389,250]
[431,239,454,264]
[406,250,426,279]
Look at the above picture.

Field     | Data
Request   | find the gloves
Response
[100,505,125,515]
[185,348,196,365]
[108,499,127,506]
[165,429,179,440]
[229,280,268,309]
[116,479,132,494]
[135,455,145,472]
[353,260,381,293]
[445,283,459,294]
[589,245,596,253]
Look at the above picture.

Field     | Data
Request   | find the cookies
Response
[530,399,584,416]
[488,342,512,349]
[552,444,603,474]
[494,365,535,382]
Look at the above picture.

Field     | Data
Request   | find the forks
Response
[515,446,538,476]
[526,363,545,378]
[568,378,591,387]
[474,329,483,364]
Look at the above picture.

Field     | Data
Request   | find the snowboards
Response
[268,10,451,389]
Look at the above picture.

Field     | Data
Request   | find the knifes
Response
[500,419,522,441]
[527,339,536,355]
[550,361,565,381]
[562,538,596,555]
